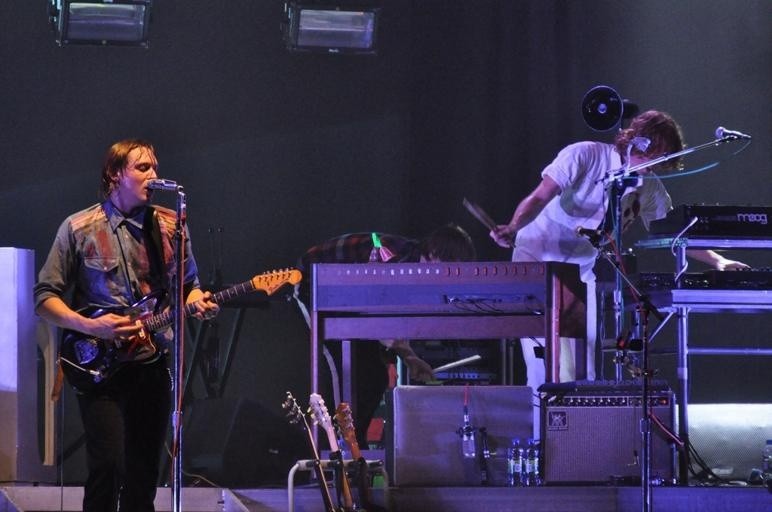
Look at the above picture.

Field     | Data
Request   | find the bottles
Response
[507,438,542,486]
[761,439,772,484]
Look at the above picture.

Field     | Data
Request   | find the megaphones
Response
[581,85,639,133]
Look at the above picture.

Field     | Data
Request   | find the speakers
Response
[538,392,675,487]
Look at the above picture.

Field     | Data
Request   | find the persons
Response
[286,231,478,451]
[489,110,750,448]
[32,137,221,512]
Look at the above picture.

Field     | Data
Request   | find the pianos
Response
[647,202,771,240]
[640,267,772,290]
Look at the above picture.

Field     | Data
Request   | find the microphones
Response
[148,179,184,192]
[715,127,751,140]
[575,226,605,238]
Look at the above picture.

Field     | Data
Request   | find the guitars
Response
[281,391,384,512]
[58,267,302,395]
